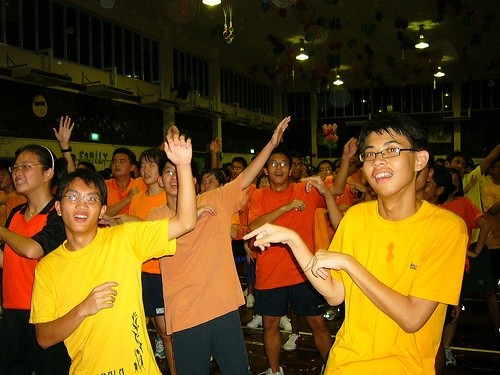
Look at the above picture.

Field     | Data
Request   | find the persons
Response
[0,115,500,375]
[243,112,469,375]
[28,126,198,375]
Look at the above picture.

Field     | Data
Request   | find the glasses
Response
[268,161,289,167]
[164,168,174,176]
[59,193,102,203]
[320,167,332,172]
[358,147,417,161]
[232,166,242,170]
[9,164,43,173]
[291,162,303,167]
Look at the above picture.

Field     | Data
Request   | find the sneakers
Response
[279,316,292,331]
[283,333,302,351]
[444,347,457,367]
[323,306,340,320]
[246,314,262,328]
[154,335,166,359]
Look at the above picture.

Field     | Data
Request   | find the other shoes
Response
[246,293,255,308]
[244,287,248,296]
[267,366,284,375]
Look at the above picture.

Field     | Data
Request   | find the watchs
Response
[60,146,73,153]
[473,249,479,255]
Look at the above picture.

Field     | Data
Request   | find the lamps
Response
[415,24,429,49]
[333,71,344,85]
[434,65,445,77]
[296,39,309,60]
